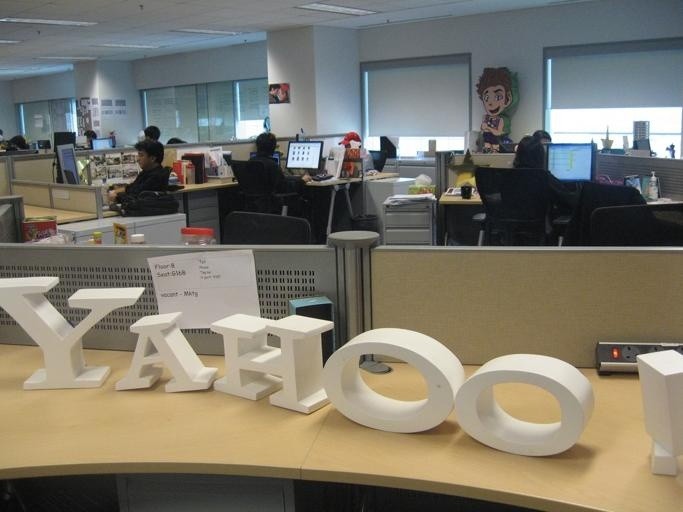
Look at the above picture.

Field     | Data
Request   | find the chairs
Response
[474,167,573,246]
[592,202,683,246]
[580,182,642,246]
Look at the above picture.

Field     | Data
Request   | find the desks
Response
[438,183,484,246]
[0,341,683,512]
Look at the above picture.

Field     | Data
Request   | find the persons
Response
[248,133,326,244]
[533,130,552,142]
[513,136,576,219]
[476,67,519,152]
[83,130,97,150]
[109,126,187,203]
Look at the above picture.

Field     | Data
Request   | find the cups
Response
[460,184,476,200]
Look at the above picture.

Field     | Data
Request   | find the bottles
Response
[178,226,215,245]
[85,230,104,244]
[130,232,148,245]
[186,163,196,186]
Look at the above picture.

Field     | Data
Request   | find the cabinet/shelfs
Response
[366,176,416,245]
[381,193,433,246]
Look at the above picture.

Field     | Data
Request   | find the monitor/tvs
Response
[285,141,323,175]
[37,140,51,149]
[602,149,625,155]
[55,144,80,184]
[499,143,519,153]
[91,137,114,150]
[633,139,652,156]
[547,143,597,183]
[54,132,76,152]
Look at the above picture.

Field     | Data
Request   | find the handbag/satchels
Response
[121,191,179,216]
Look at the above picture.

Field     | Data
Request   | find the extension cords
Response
[595,342,683,374]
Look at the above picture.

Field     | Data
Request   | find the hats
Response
[338,132,361,145]
